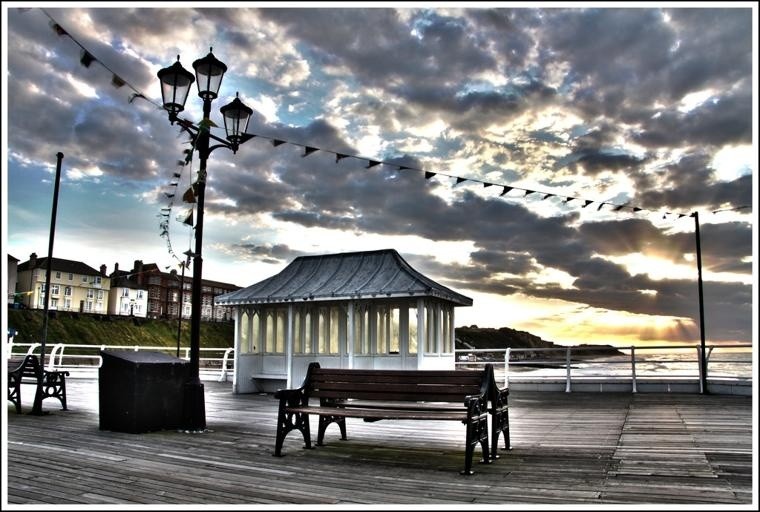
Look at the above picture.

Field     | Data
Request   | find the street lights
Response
[158,45,255,431]
[26,252,38,309]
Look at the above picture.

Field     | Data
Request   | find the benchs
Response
[272,361,513,477]
[8,354,70,417]
